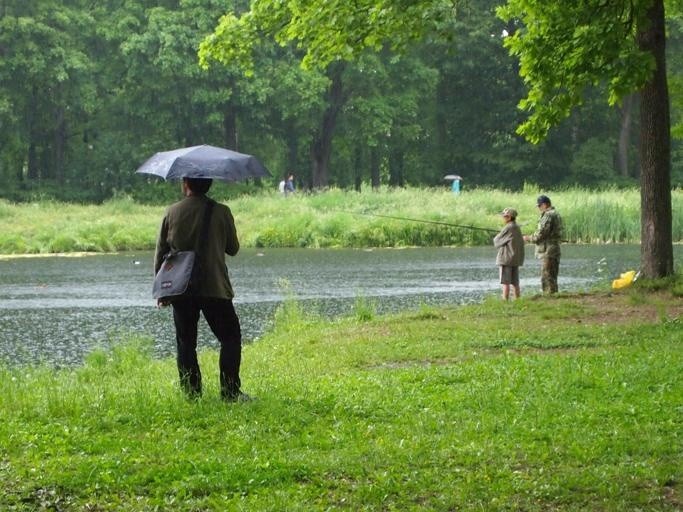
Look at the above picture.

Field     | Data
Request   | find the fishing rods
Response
[334,210,569,243]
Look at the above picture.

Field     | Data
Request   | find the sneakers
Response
[220,388,259,403]
[186,388,203,402]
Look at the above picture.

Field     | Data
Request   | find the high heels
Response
[151,249,199,299]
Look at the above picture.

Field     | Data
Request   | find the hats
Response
[535,195,550,206]
[499,207,518,219]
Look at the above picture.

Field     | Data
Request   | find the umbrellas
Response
[444,174,463,181]
[134,143,276,183]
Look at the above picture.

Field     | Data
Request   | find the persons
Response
[522,194,565,295]
[451,179,461,193]
[278,178,286,194]
[493,208,525,301]
[153,176,259,404]
[283,172,298,195]
